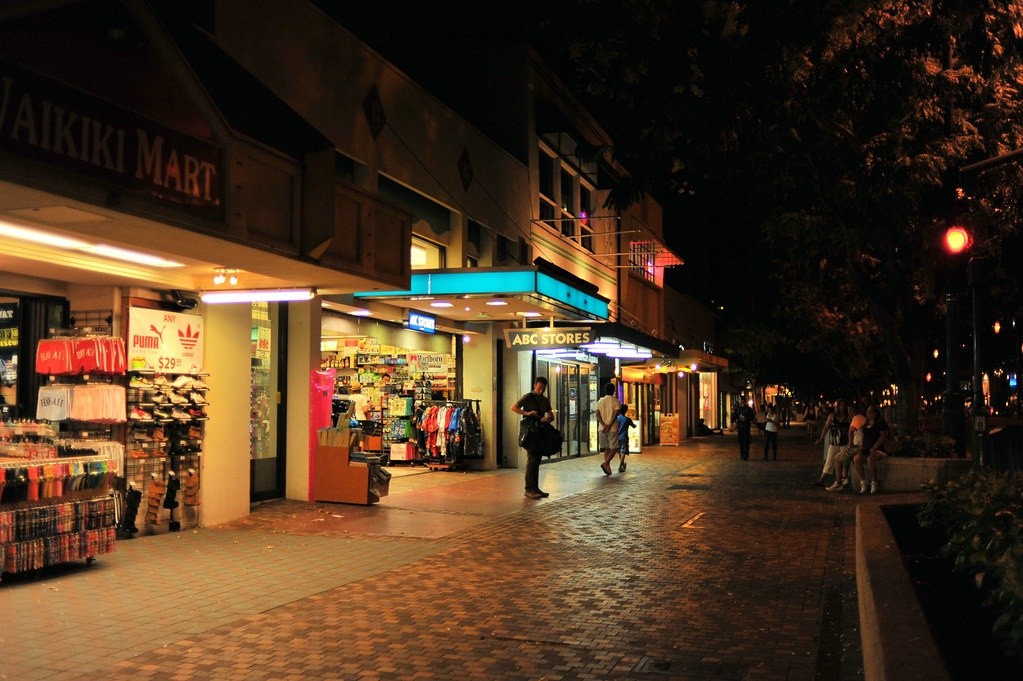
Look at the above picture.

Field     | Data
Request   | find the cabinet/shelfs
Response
[127,370,209,449]
[321,352,456,401]
[313,446,379,504]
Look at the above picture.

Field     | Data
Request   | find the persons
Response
[815,399,890,493]
[802,401,828,439]
[595,383,620,474]
[382,373,391,382]
[617,404,636,472]
[756,405,767,440]
[763,405,778,461]
[731,396,754,460]
[512,377,554,497]
[350,380,370,451]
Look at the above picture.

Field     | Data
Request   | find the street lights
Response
[943,224,982,473]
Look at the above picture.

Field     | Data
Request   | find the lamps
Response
[656,357,671,371]
[198,287,317,305]
[213,266,238,285]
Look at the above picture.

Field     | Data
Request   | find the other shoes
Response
[860,480,867,493]
[618,468,625,472]
[841,478,850,486]
[825,481,844,491]
[813,480,824,486]
[536,489,549,497]
[623,462,627,470]
[129,374,210,460]
[601,462,612,475]
[524,491,541,498]
[871,480,878,494]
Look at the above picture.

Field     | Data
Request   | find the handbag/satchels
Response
[882,432,899,454]
